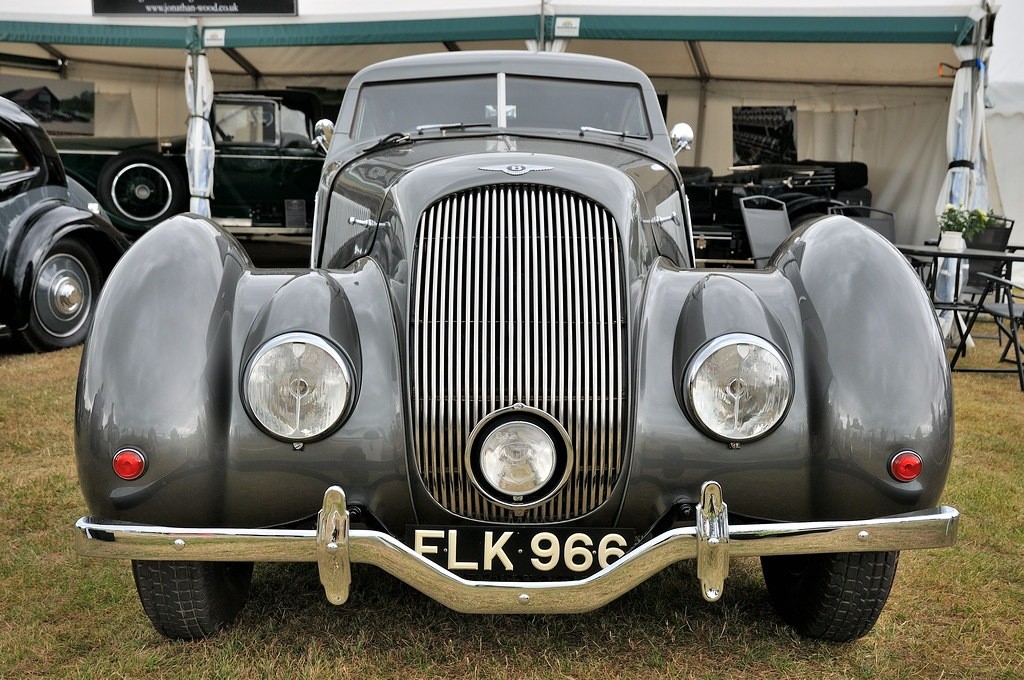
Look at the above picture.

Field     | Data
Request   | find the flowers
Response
[937,202,997,243]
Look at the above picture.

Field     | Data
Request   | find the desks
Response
[896,243,1024,347]
[226,226,312,240]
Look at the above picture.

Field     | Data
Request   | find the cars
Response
[72,48,961,645]
[1,82,324,260]
[0,94,131,356]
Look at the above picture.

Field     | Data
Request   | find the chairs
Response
[962,215,1015,322]
[950,271,1024,392]
[739,195,792,269]
[827,206,897,245]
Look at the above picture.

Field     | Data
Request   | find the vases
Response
[939,231,967,248]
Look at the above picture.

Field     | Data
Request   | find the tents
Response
[0,0,1024,351]
